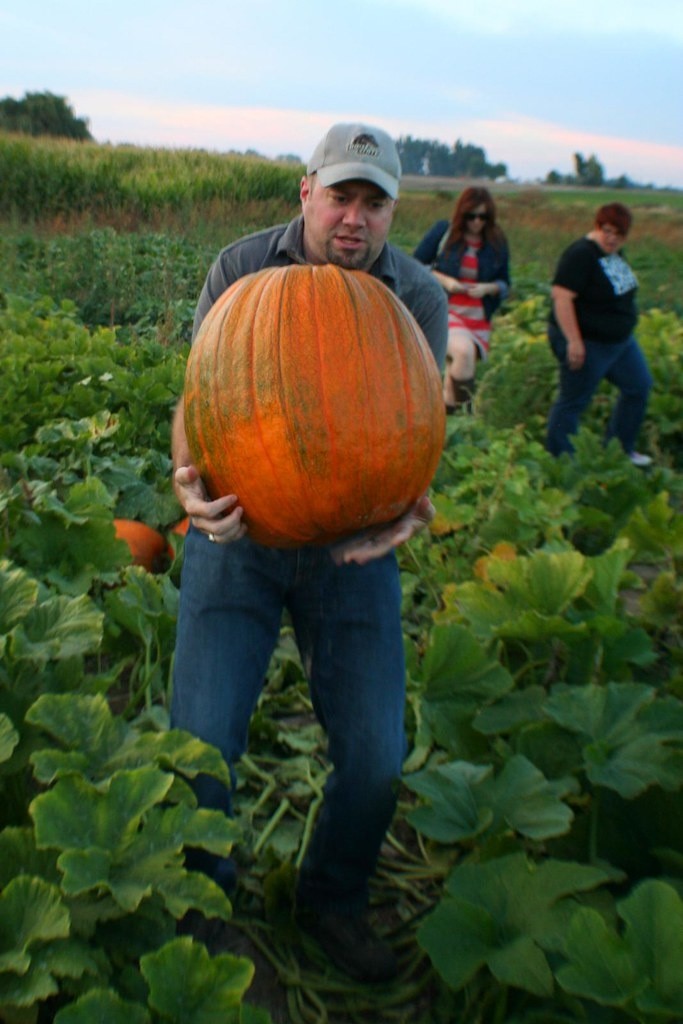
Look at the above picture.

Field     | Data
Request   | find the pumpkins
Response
[112,517,192,573]
[182,263,446,549]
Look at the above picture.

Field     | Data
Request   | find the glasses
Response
[600,225,624,237]
[464,212,489,222]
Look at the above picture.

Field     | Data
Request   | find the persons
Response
[152,121,449,997]
[412,185,511,417]
[545,203,655,467]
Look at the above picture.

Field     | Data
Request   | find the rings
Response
[366,534,381,545]
[208,531,215,543]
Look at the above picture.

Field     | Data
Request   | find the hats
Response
[306,123,403,200]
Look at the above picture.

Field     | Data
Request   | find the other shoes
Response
[174,912,275,1004]
[628,450,654,466]
[294,894,400,987]
